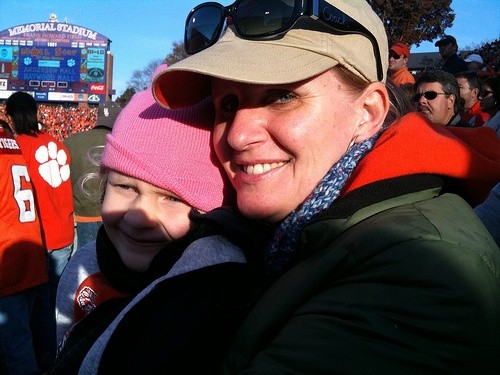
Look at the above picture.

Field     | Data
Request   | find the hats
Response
[101,63,228,213]
[390,43,410,58]
[435,35,456,46]
[92,99,122,131]
[152,0,388,111]
[463,55,483,64]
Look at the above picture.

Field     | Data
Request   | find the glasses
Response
[479,90,494,98]
[391,54,400,58]
[184,0,384,82]
[412,90,451,101]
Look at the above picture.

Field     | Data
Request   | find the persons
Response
[1,120,60,374]
[477,76,500,139]
[412,69,475,130]
[61,100,124,255]
[0,100,98,142]
[454,70,491,129]
[480,62,500,76]
[435,34,468,74]
[152,0,500,375]
[386,41,417,86]
[3,91,75,313]
[54,61,260,375]
[465,53,488,77]
